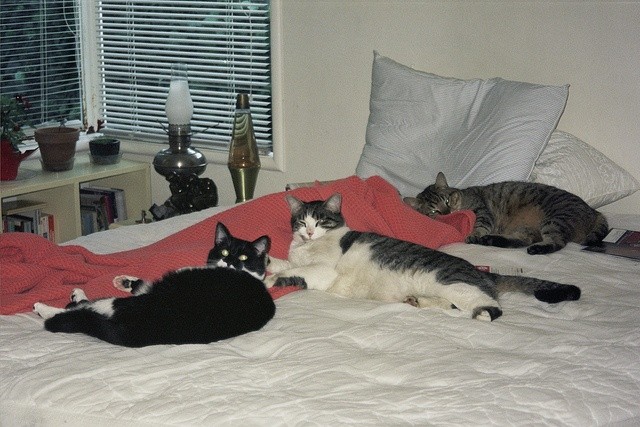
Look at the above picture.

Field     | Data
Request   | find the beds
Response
[1,188,637,427]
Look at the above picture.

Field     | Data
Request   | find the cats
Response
[403,172,609,256]
[264,194,581,322]
[32,221,276,348]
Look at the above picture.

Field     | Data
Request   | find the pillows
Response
[349,48,572,201]
[528,131,640,209]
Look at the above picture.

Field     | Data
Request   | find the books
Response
[80,186,128,236]
[1,210,56,242]
[2,200,48,215]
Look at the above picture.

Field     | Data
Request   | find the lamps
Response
[145,61,210,221]
[224,92,262,206]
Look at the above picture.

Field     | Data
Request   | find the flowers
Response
[0,93,37,151]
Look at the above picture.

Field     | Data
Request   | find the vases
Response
[88,139,122,163]
[34,126,81,172]
[0,136,35,182]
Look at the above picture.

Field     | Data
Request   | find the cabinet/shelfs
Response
[2,160,154,245]
